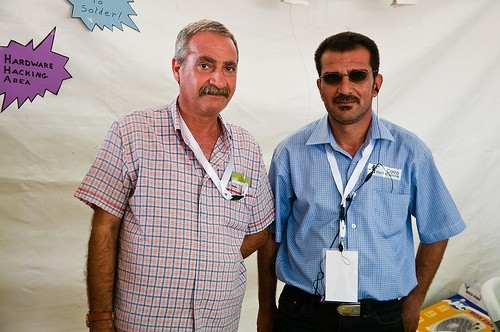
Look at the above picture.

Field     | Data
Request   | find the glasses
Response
[320,70,377,83]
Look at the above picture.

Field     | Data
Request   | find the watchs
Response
[85,311,114,327]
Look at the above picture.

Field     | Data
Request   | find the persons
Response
[74,19,276,332]
[256,32,467,332]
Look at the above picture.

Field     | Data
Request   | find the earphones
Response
[375,88,379,93]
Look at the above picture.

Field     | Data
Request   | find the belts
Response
[284,285,400,317]
